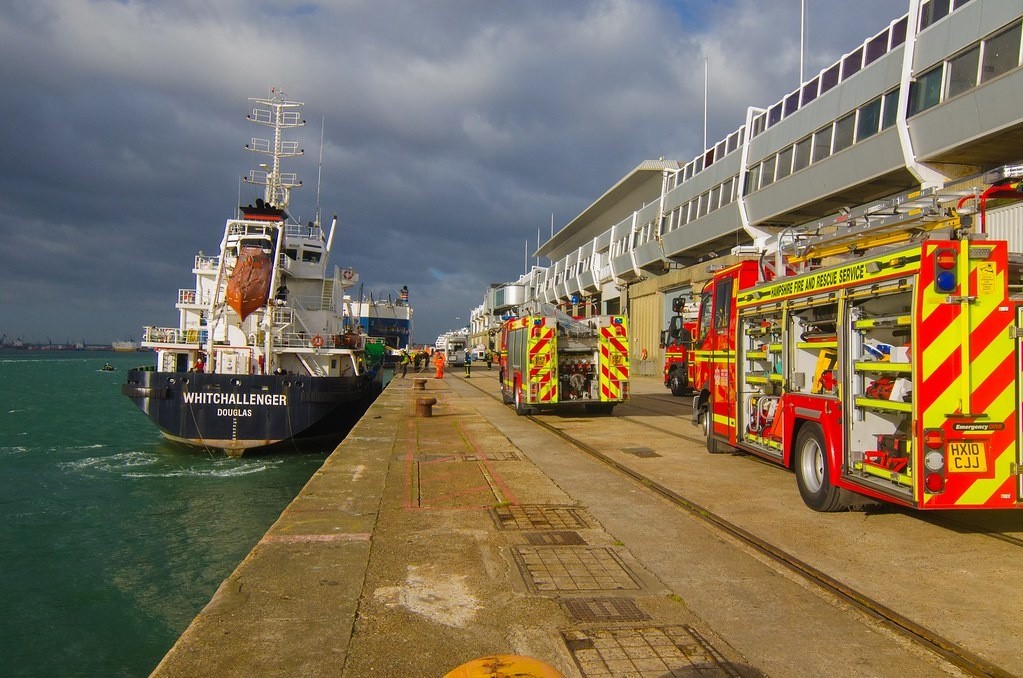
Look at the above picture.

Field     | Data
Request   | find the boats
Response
[100,361,118,374]
[122,81,387,461]
[112,335,142,352]
[343,286,414,370]
[434,325,469,352]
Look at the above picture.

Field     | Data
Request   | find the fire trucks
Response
[490,297,630,416]
[657,166,1023,512]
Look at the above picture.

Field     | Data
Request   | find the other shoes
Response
[464,376,470,378]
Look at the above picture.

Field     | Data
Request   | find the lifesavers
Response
[370,338,376,344]
[311,335,325,347]
[343,270,353,280]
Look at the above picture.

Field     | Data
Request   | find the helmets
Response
[464,348,468,352]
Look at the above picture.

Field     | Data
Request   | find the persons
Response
[401,350,430,378]
[432,350,444,378]
[194,357,204,372]
[464,348,472,378]
[274,367,288,374]
[486,349,492,371]
